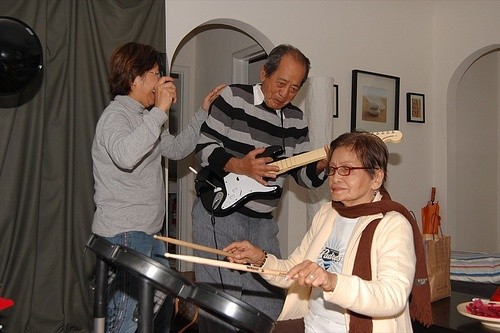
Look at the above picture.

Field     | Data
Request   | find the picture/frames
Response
[332,85,339,117]
[406,92,426,123]
[351,70,400,140]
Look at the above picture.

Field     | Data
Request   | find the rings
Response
[309,274,315,280]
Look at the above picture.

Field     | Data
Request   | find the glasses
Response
[324,166,378,176]
[149,71,164,78]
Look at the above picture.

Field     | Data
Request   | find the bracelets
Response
[254,250,268,267]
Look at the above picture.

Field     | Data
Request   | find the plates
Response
[457,299,500,332]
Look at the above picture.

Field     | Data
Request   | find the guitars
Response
[195,129,404,218]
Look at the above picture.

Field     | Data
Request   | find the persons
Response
[91,42,226,333]
[191,44,330,333]
[222,129,417,333]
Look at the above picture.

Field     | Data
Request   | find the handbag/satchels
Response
[408,210,452,303]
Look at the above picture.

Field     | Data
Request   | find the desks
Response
[408,290,495,333]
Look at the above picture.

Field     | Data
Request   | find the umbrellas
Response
[421,187,441,235]
